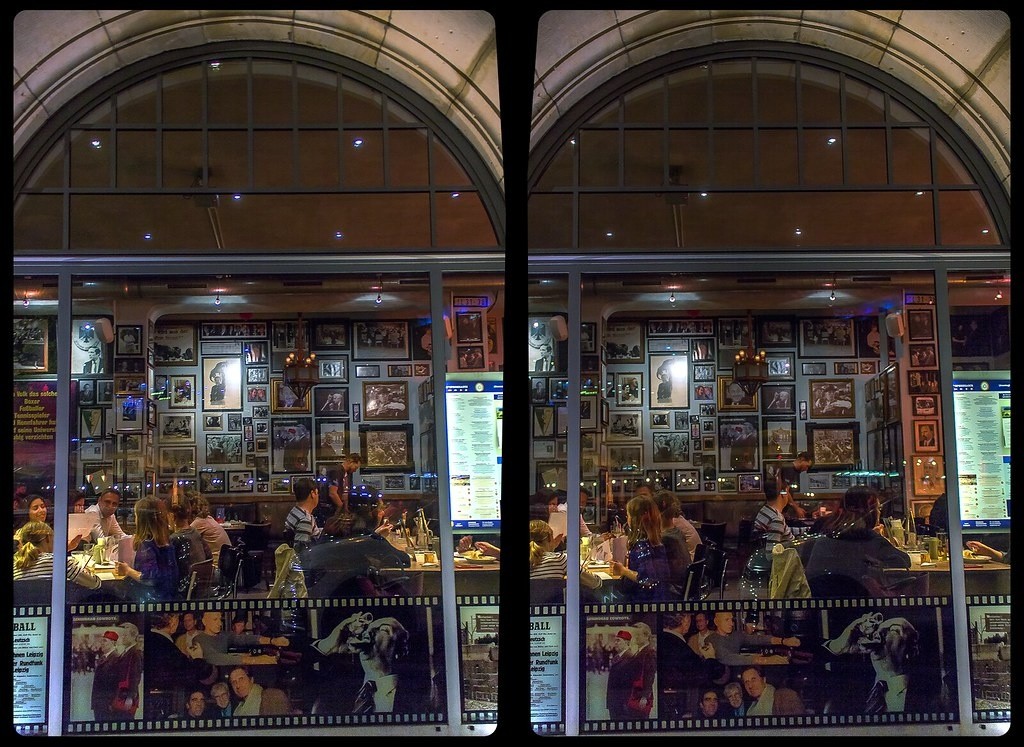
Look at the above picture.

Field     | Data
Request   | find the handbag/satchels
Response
[112,681,139,717]
[627,680,653,715]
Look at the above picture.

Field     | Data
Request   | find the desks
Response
[882,548,1011,596]
[370,536,499,596]
[223,527,246,547]
[69,556,143,593]
[564,563,621,599]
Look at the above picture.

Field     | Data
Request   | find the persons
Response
[73,623,142,722]
[610,481,702,602]
[146,612,293,715]
[308,611,428,713]
[455,488,602,603]
[820,614,933,714]
[287,454,410,598]
[587,622,656,719]
[931,493,1010,564]
[659,613,807,713]
[13,480,231,602]
[755,452,912,598]
[163,325,431,474]
[81,332,136,402]
[609,322,893,473]
[530,343,555,401]
[908,316,935,446]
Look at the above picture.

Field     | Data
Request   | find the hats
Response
[104,631,118,641]
[616,631,632,641]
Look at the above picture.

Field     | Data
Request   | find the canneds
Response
[928,537,939,559]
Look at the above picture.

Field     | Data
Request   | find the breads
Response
[424,553,436,564]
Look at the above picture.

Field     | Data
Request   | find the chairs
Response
[117,501,893,717]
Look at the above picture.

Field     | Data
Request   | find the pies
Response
[466,549,486,560]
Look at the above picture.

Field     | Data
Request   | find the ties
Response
[862,680,888,713]
[351,680,377,714]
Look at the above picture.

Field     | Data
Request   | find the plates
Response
[454,552,483,557]
[465,556,497,563]
[964,555,992,562]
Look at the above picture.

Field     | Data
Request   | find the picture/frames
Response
[12,304,945,536]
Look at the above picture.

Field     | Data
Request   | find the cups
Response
[72,544,95,563]
[414,550,434,564]
[908,550,930,567]
[216,508,225,524]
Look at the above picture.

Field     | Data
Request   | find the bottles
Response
[580,537,591,560]
[95,538,107,562]
[929,537,939,559]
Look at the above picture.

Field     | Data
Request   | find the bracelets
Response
[270,638,273,644]
[781,637,784,645]
